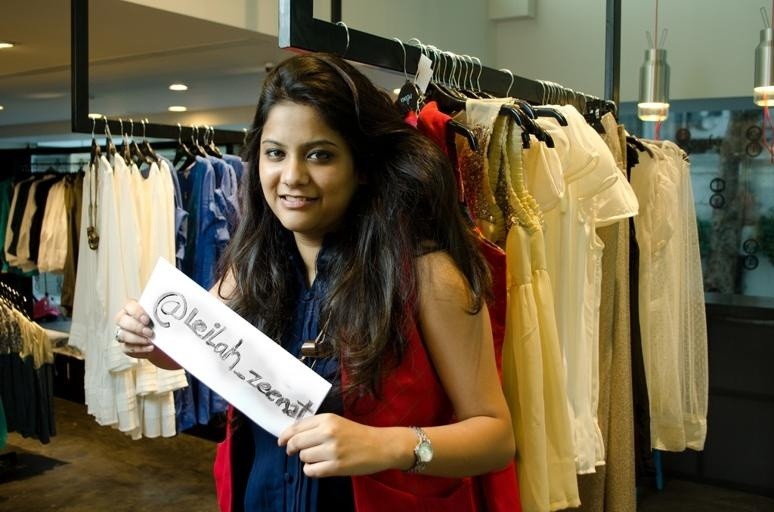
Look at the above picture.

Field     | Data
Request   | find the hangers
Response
[325,18,694,167]
[23,157,83,177]
[0,280,36,322]
[80,113,228,164]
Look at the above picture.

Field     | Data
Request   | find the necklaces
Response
[87,147,100,249]
[300,310,332,371]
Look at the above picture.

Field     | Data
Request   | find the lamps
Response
[752,18,774,110]
[637,1,672,124]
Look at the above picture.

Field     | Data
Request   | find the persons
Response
[114,52,517,512]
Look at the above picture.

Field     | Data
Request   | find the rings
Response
[115,330,123,343]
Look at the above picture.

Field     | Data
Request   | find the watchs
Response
[405,426,434,473]
